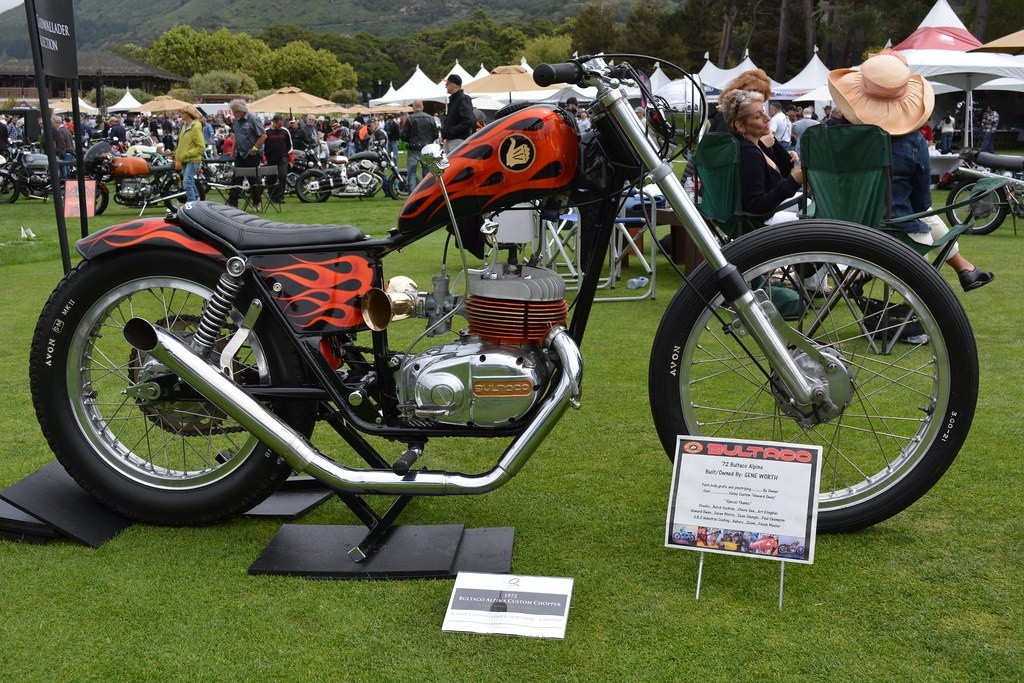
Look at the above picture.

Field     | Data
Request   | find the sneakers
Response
[958,267,995,292]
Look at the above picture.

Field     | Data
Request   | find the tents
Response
[648,36,892,118]
[860,0,1024,153]
[368,49,642,113]
[51,82,151,115]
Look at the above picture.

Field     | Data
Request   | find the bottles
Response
[697,176,702,204]
[665,200,673,211]
[627,277,649,290]
[684,177,695,204]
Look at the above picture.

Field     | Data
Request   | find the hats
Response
[441,74,462,85]
[828,54,935,135]
[318,116,326,122]
[385,114,395,119]
[803,108,812,118]
[566,97,578,106]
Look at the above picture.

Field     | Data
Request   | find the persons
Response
[403,74,476,196]
[0,100,402,217]
[564,96,593,136]
[679,51,1000,301]
[634,103,650,129]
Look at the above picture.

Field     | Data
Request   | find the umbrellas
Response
[459,57,573,104]
[44,92,193,114]
[966,30,1024,57]
[245,82,414,120]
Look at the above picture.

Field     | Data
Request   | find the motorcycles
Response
[30,51,981,535]
[0,100,418,215]
[945,146,1024,235]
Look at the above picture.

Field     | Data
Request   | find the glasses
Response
[733,91,748,121]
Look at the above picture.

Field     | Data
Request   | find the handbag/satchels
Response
[861,294,927,343]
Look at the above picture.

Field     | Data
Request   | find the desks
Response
[609,207,717,278]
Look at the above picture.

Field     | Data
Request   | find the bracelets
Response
[252,146,259,152]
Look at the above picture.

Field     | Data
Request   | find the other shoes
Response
[280,199,286,204]
[251,202,262,213]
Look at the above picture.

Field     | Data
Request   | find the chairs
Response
[798,124,1007,355]
[206,164,281,215]
[542,186,656,302]
[691,132,810,320]
